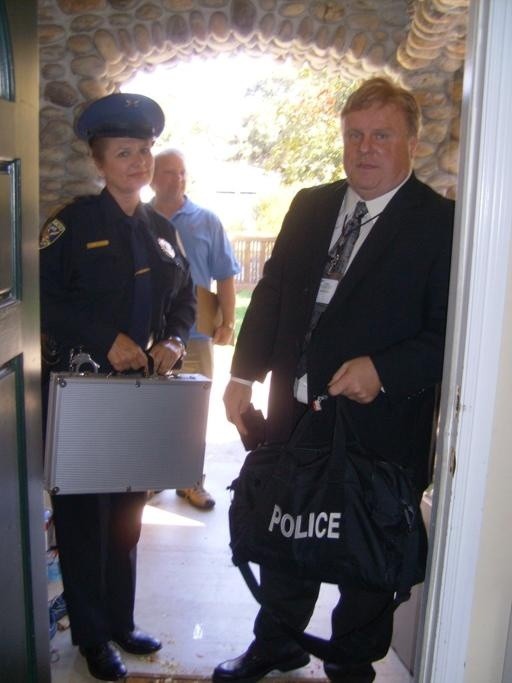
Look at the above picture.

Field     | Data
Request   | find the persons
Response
[146,148,243,509]
[40,92,198,681]
[211,77,455,683]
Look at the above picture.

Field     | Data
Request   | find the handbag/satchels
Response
[228,391,428,587]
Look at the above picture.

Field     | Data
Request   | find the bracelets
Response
[164,335,186,360]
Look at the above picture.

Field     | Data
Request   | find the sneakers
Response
[176,478,215,508]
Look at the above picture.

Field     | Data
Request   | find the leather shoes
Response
[79,642,127,680]
[212,638,310,682]
[111,628,161,654]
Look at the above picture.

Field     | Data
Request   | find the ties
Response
[124,216,152,346]
[295,201,368,380]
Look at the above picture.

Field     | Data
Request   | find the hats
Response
[75,93,165,142]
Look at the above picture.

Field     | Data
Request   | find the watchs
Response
[223,319,236,329]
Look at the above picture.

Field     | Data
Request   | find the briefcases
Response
[45,355,212,496]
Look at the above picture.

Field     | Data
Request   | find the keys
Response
[327,259,337,276]
